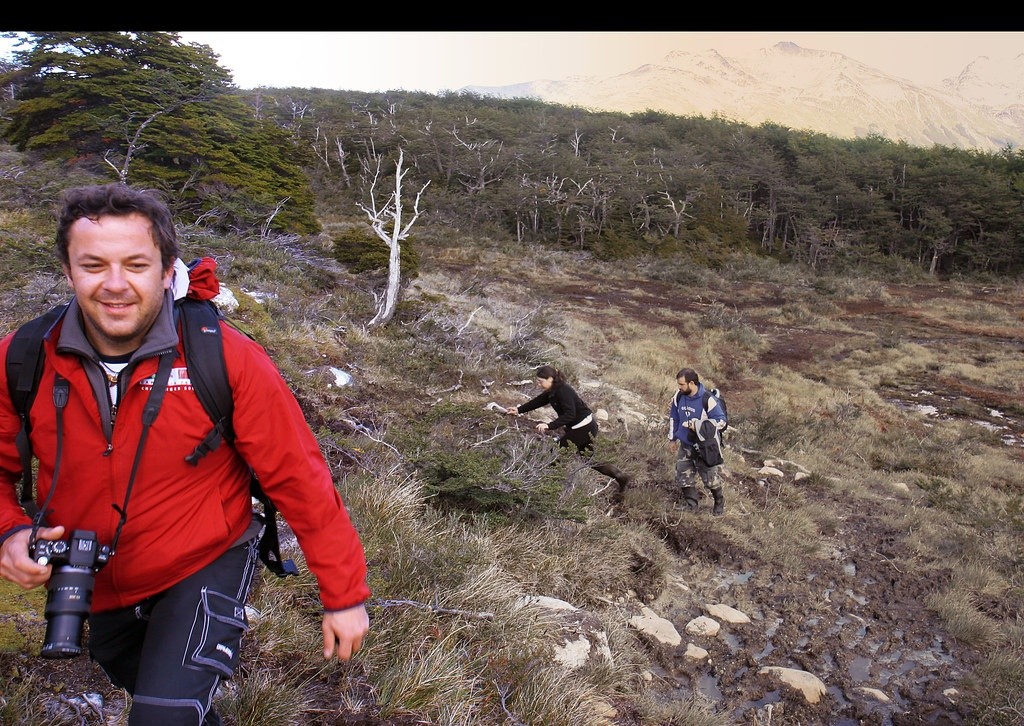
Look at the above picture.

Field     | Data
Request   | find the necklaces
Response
[684,394,696,428]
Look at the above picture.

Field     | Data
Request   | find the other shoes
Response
[618,473,633,494]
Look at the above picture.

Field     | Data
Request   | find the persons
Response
[667,367,728,515]
[507,366,598,456]
[0,184,369,726]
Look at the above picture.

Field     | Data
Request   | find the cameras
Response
[28,530,110,660]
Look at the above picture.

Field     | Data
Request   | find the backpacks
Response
[676,388,728,434]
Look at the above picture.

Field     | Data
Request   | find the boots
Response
[681,486,699,514]
[711,487,725,516]
